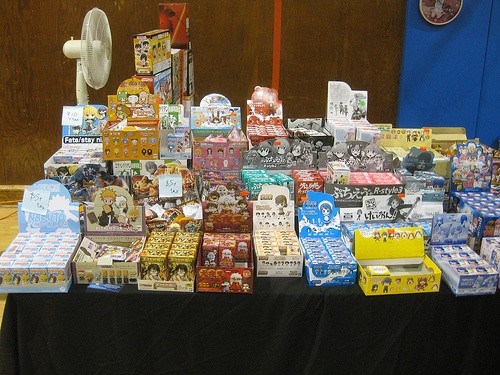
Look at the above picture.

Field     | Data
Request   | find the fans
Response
[62,6,113,106]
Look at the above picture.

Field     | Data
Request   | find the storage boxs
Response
[116,1,500,296]
[0,78,160,294]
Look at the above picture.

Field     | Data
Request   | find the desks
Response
[0,283,500,375]
